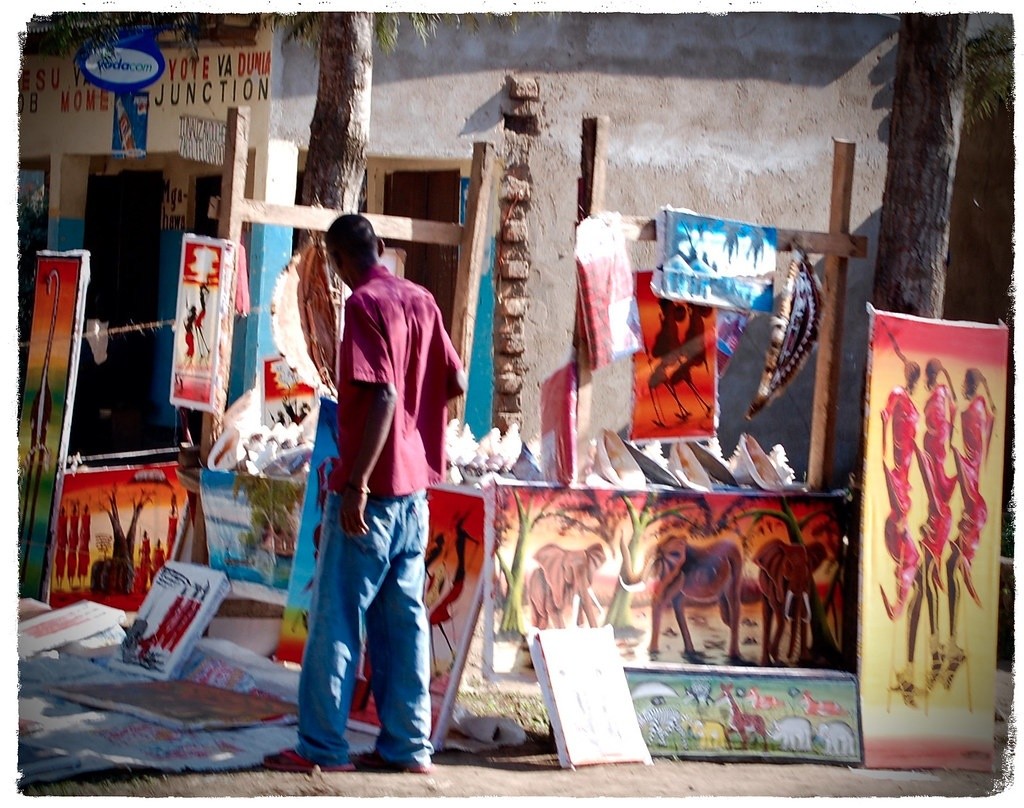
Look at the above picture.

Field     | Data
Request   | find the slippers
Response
[350,749,430,774]
[264,747,356,771]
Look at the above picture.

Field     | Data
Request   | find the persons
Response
[264,215,468,771]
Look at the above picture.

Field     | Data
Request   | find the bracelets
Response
[348,482,372,495]
